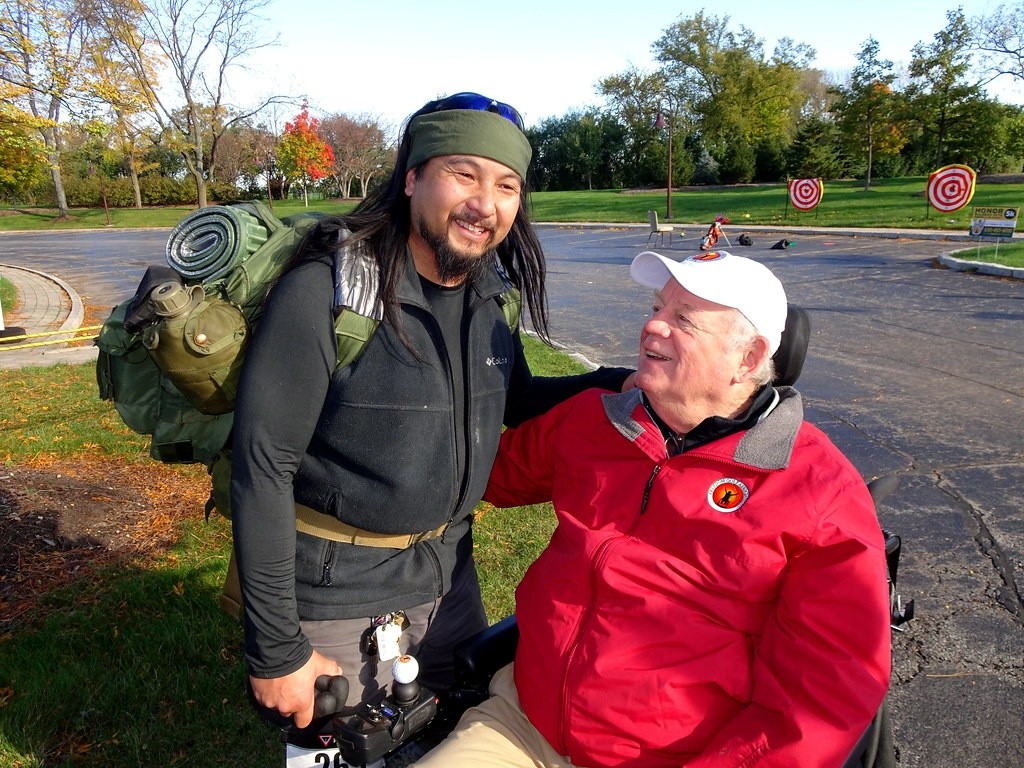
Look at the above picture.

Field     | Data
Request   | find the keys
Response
[365,617,404,657]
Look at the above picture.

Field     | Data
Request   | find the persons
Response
[404,253,891,768]
[228,93,634,768]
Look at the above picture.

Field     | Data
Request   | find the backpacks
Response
[93,199,522,548]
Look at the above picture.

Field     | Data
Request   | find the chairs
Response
[647,210,674,248]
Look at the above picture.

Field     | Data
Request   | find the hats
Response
[630,250,787,359]
